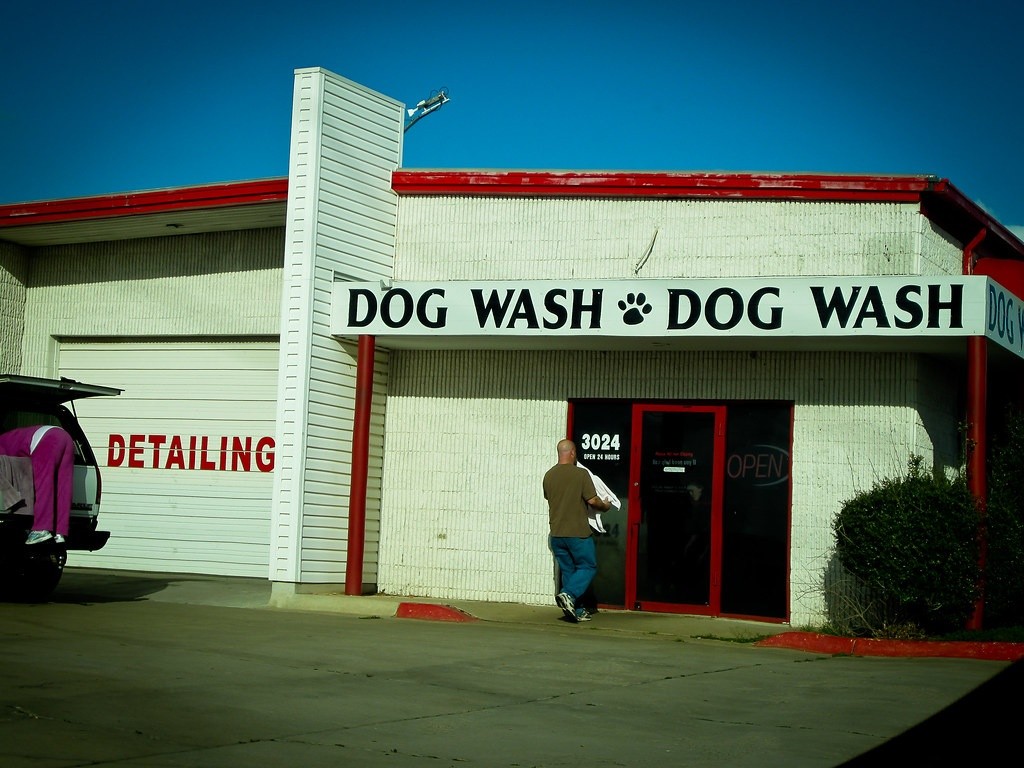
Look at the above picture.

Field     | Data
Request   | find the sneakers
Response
[25,529,52,544]
[578,609,592,621]
[53,534,65,544]
[556,593,578,622]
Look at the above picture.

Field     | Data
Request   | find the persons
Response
[0,425,75,544]
[544,439,611,622]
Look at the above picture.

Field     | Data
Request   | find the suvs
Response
[0,373,125,603]
